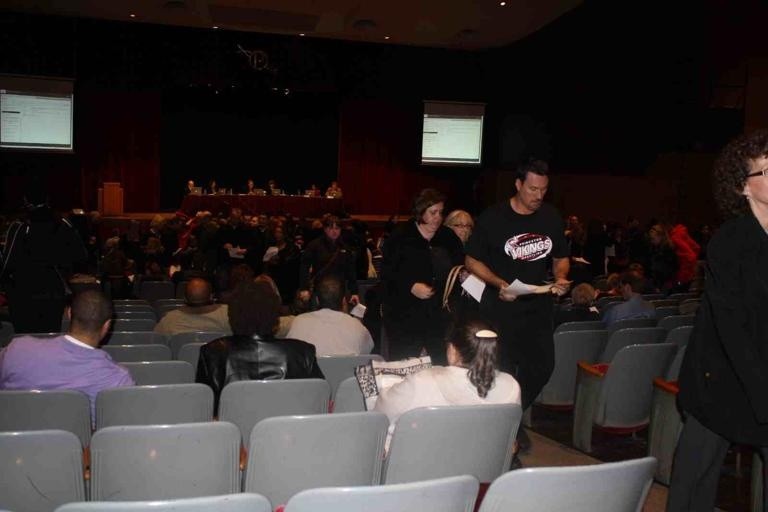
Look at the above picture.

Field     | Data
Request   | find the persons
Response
[195,279,326,421]
[664,125,767,512]
[443,316,525,512]
[442,209,477,310]
[2,289,137,427]
[333,284,437,458]
[465,158,574,450]
[376,187,464,366]
[555,212,724,328]
[1,179,414,356]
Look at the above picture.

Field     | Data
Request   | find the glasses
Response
[452,223,472,230]
[745,168,768,178]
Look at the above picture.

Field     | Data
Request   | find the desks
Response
[184,193,345,216]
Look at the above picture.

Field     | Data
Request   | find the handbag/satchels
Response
[438,296,478,329]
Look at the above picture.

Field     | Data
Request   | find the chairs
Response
[0,263,768,512]
